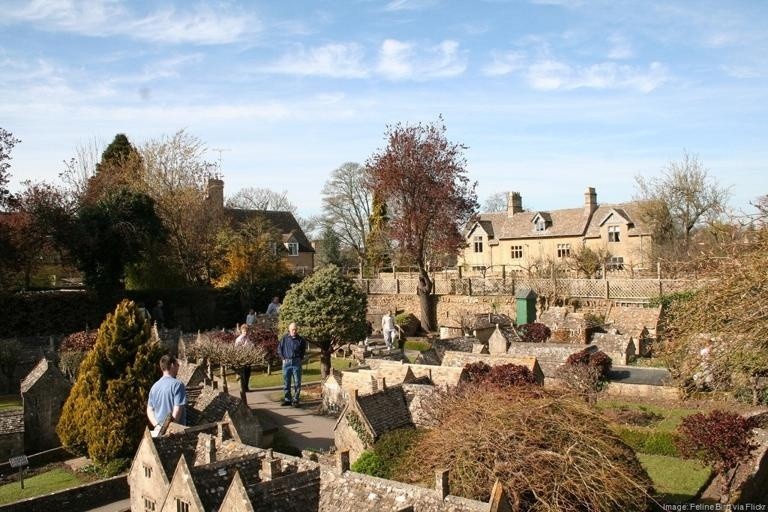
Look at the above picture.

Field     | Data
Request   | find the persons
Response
[688,339,716,392]
[144,353,189,428]
[277,322,307,408]
[381,309,397,350]
[234,322,257,392]
[245,308,257,325]
[266,296,282,316]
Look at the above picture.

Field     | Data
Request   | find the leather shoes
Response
[282,401,291,406]
[294,402,300,408]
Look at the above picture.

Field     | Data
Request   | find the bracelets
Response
[153,423,159,428]
